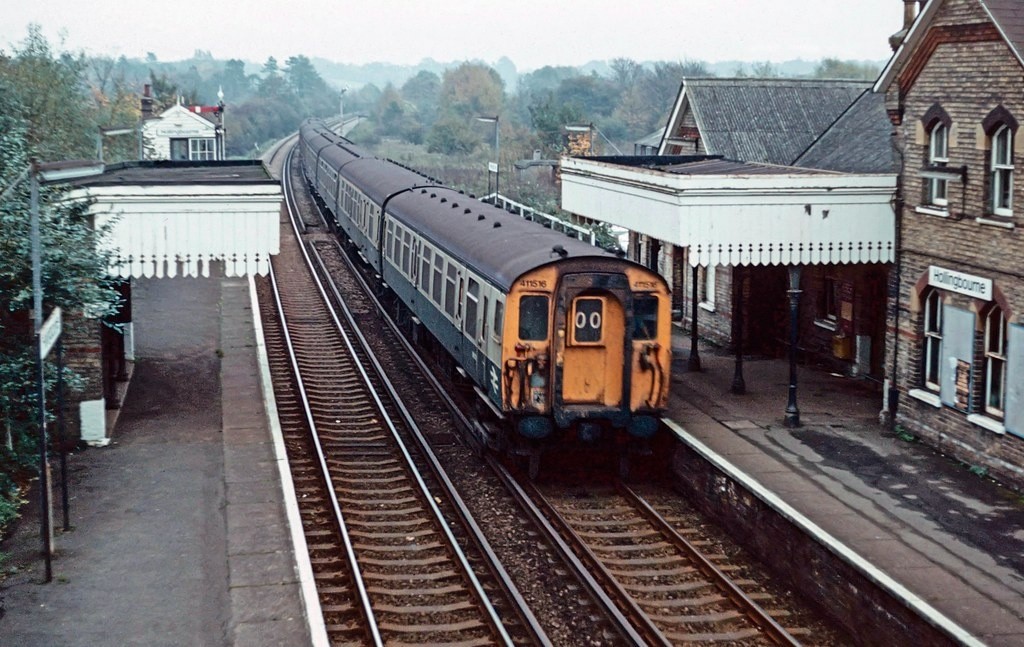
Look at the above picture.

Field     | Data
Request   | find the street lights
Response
[476,115,499,206]
[30,159,105,335]
[137,116,163,161]
[565,122,594,157]
[97,124,134,163]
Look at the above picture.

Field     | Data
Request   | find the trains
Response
[299,117,674,470]
